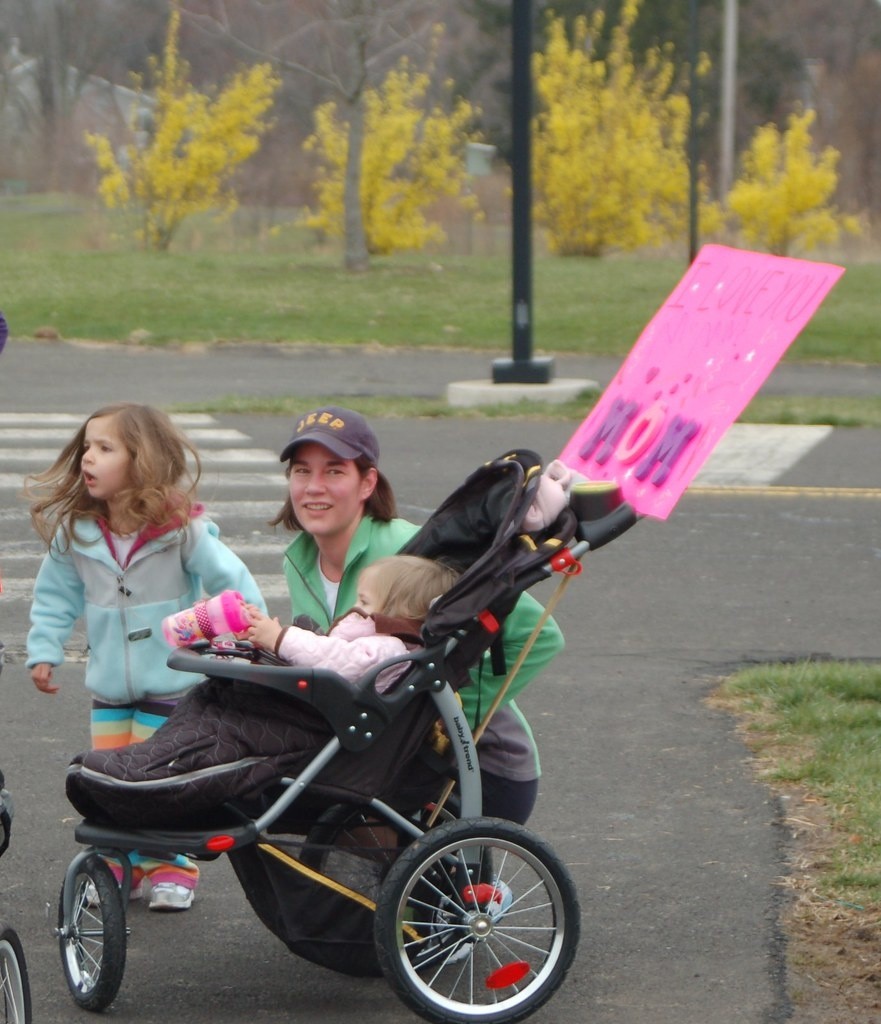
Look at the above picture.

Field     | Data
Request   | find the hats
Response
[280,406,379,467]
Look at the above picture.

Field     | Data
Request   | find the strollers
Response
[0,764,34,1024]
[52,444,647,1024]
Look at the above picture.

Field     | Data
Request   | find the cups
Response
[162,589,252,648]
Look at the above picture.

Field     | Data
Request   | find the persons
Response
[25,402,270,912]
[233,553,461,697]
[273,406,565,970]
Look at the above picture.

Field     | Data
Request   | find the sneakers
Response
[417,873,514,966]
[148,882,194,910]
[85,882,143,906]
